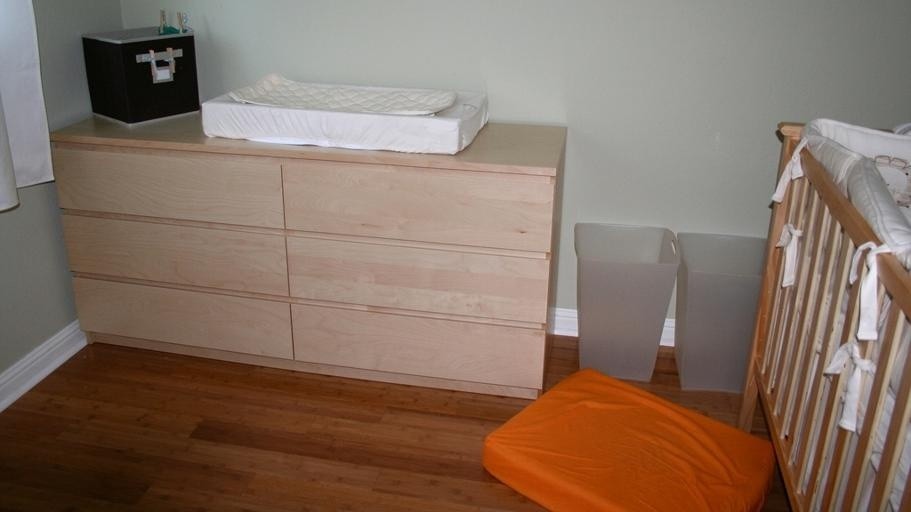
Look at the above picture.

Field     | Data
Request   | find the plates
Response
[45,110,570,405]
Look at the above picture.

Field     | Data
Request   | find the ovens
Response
[734,121,911,511]
